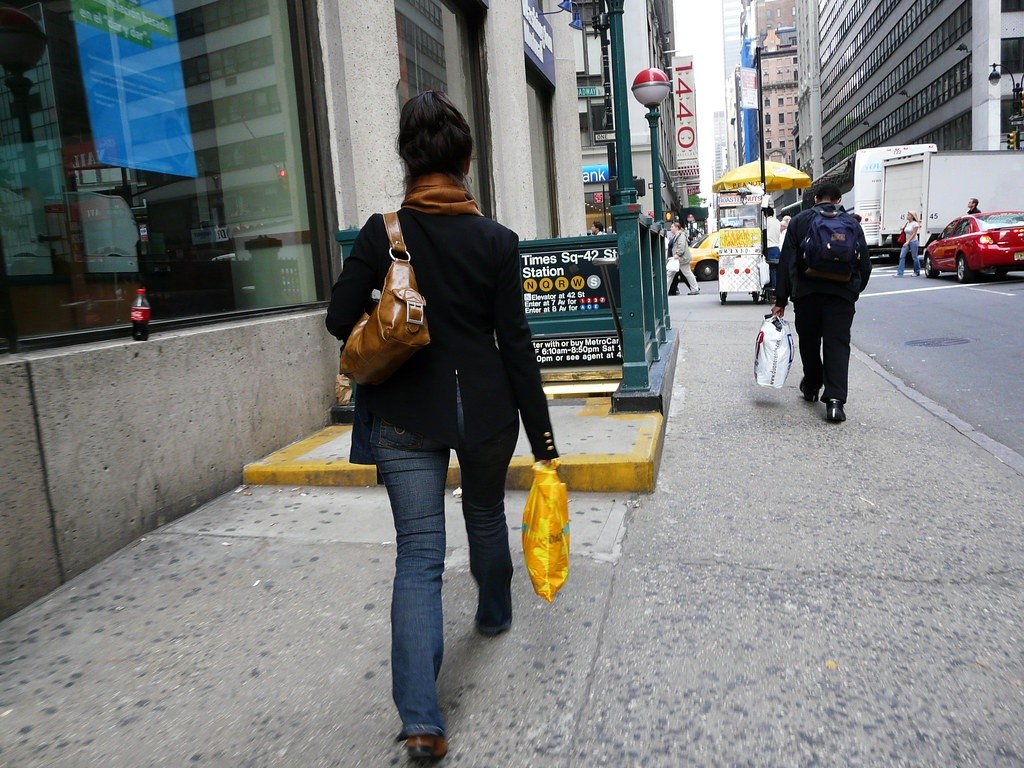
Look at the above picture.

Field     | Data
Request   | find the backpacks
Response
[799,206,859,283]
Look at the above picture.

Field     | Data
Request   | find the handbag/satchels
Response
[522,460,570,601]
[340,211,430,386]
[667,257,679,272]
[897,223,908,243]
[754,314,794,389]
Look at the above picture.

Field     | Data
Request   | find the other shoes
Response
[406,735,447,759]
[911,273,918,276]
[688,291,699,295]
[804,392,819,401]
[894,274,902,277]
[826,400,846,420]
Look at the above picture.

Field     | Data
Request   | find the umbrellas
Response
[712,160,811,193]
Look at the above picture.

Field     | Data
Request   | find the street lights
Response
[0,5,49,236]
[988,62,1024,149]
[631,68,671,229]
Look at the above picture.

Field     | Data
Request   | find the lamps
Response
[863,120,870,127]
[956,43,972,53]
[820,156,826,161]
[899,90,910,97]
[838,141,845,147]
[537,0,584,30]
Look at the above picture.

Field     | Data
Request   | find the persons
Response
[761,206,793,290]
[966,197,982,214]
[591,220,607,237]
[771,181,872,423]
[893,210,921,279]
[665,223,701,295]
[325,89,562,762]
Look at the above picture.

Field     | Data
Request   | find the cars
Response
[686,231,719,280]
[923,211,1024,283]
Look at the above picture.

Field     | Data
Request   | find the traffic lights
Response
[1006,131,1017,151]
[1019,91,1024,116]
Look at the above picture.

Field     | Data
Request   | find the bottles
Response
[131,288,150,340]
